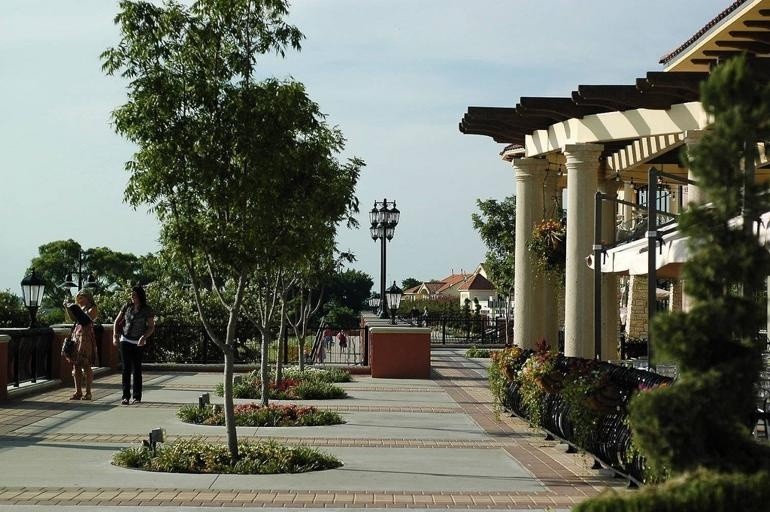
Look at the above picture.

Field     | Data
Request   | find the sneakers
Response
[121,398,140,405]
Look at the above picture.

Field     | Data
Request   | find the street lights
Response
[57,250,100,292]
[369,199,403,325]
[21,265,46,383]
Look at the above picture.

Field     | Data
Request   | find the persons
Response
[76,289,97,321]
[323,325,333,353]
[336,329,346,353]
[112,286,155,405]
[65,305,98,400]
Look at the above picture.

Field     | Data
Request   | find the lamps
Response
[548,162,676,202]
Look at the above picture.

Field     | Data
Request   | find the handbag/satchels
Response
[114,318,126,349]
[62,338,74,357]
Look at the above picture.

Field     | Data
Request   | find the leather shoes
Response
[69,392,91,400]
